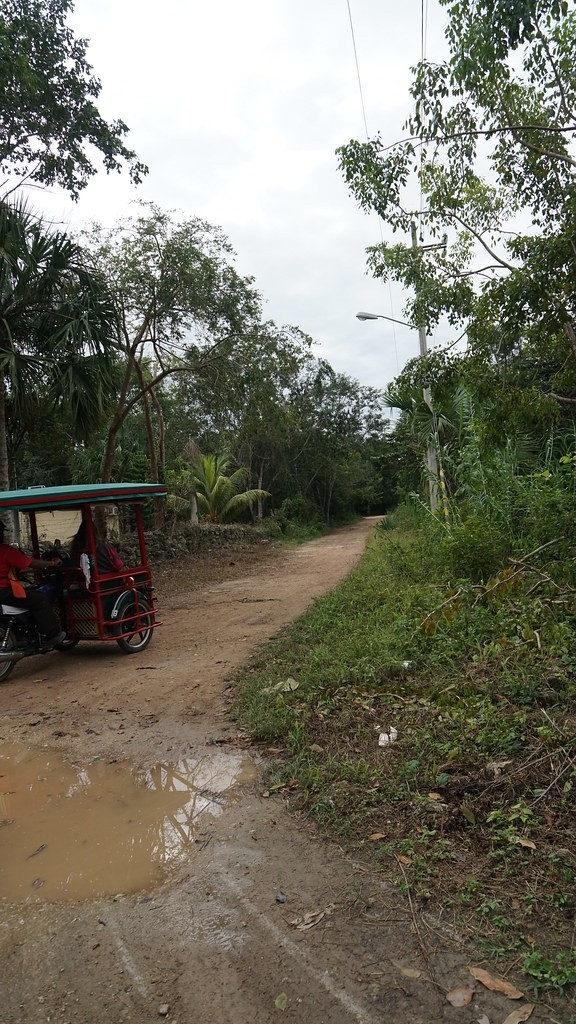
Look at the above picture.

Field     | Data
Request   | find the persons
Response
[0,521,66,643]
[68,519,123,574]
[47,549,61,561]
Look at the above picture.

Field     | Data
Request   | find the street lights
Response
[356,311,441,520]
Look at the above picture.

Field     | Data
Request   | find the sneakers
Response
[40,630,66,650]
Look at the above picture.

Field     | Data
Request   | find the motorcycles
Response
[0,482,171,684]
